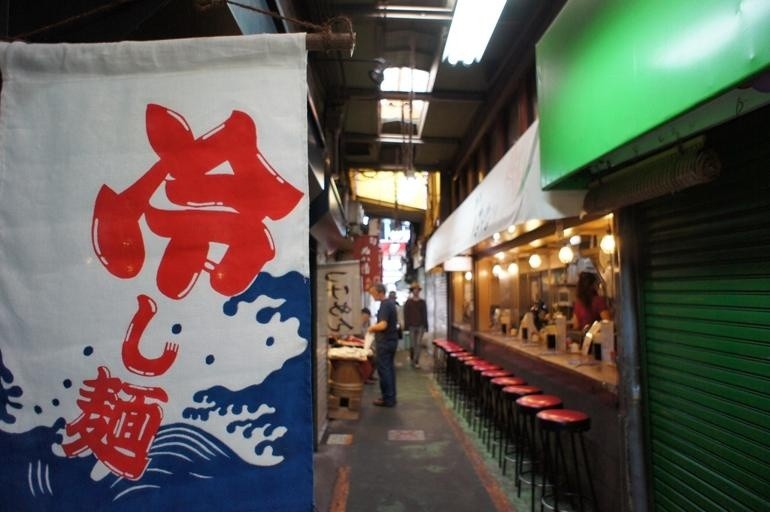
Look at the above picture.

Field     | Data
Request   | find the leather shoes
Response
[374,399,396,407]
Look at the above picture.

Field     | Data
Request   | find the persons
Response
[405,283,431,369]
[388,290,405,341]
[368,283,399,409]
[571,273,613,333]
[346,307,371,343]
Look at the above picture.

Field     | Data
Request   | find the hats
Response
[409,282,422,289]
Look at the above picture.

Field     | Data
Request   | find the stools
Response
[431,338,598,510]
